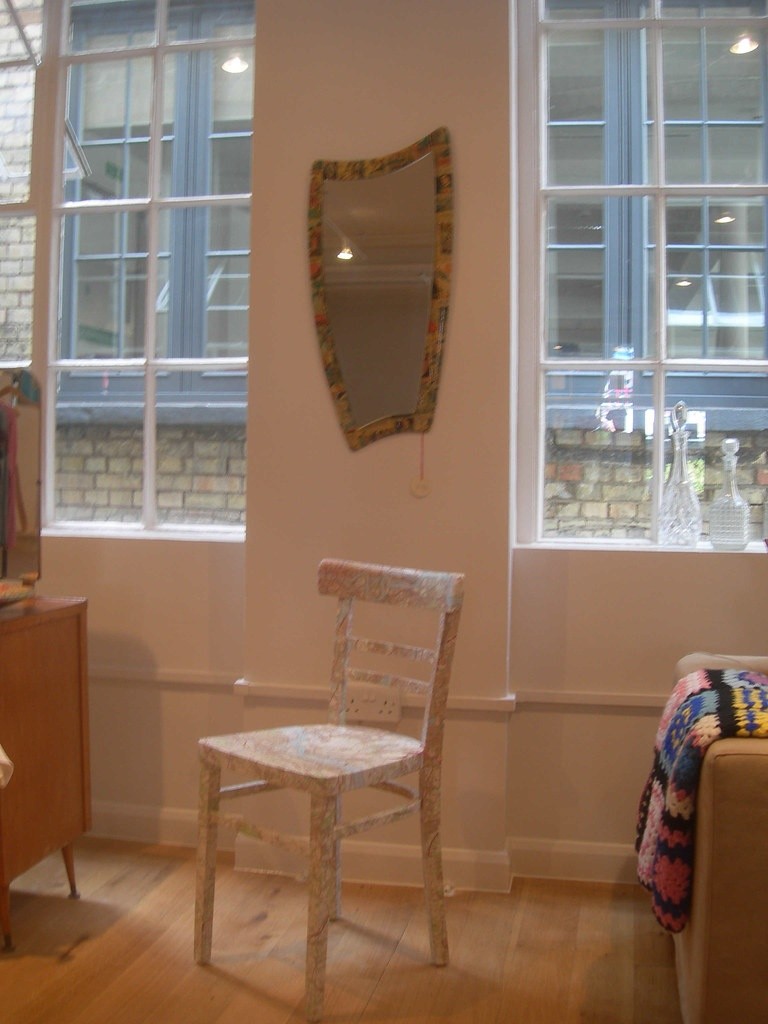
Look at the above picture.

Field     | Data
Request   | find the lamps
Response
[729,30,761,54]
[222,46,249,74]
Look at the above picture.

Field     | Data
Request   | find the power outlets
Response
[344,682,403,724]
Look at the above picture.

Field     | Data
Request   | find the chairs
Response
[191,557,464,1023]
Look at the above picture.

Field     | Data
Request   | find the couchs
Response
[668,654,768,1024]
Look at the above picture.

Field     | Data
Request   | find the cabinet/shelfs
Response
[1,589,92,953]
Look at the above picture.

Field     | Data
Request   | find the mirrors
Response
[307,126,452,453]
